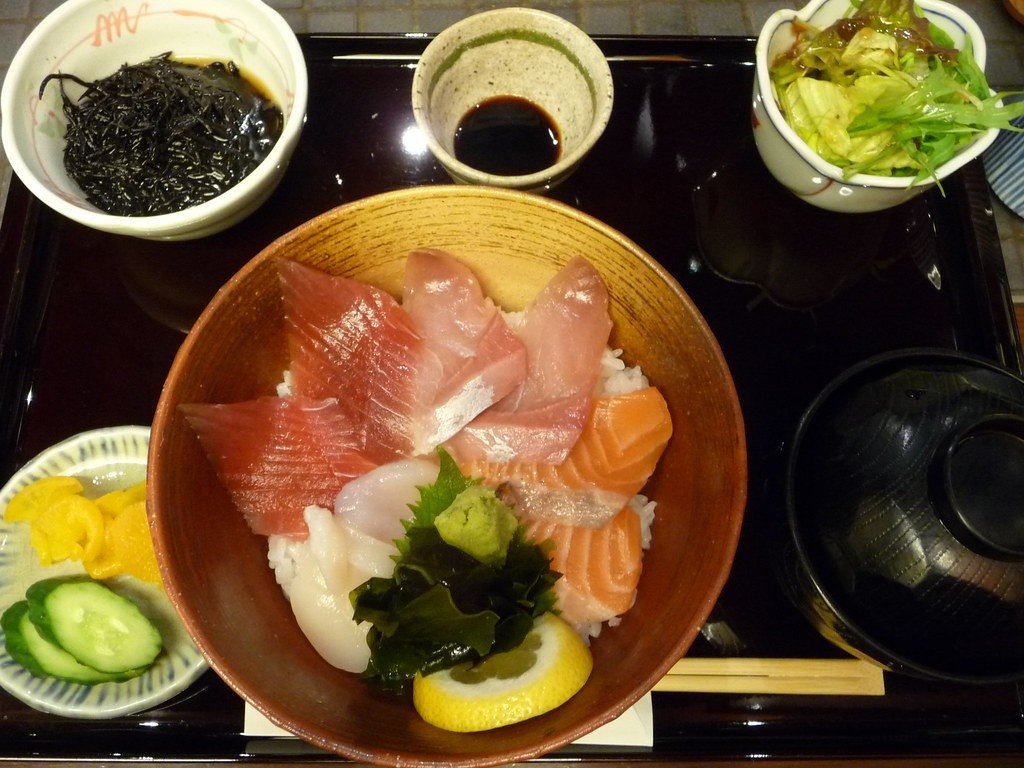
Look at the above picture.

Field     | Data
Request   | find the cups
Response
[412,5,615,193]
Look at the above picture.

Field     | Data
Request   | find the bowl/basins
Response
[751,0,1007,213]
[0,0,310,241]
[144,189,746,768]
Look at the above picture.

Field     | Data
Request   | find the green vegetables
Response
[766,0,1024,199]
[350,445,560,691]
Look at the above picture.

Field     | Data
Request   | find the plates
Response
[0,424,204,720]
[788,352,1024,685]
[944,412,1024,560]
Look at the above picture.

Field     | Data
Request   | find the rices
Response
[265,313,656,640]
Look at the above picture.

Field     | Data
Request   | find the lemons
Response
[409,612,592,732]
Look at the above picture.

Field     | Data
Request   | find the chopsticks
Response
[652,657,884,695]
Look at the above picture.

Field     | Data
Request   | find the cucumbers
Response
[0,576,167,685]
